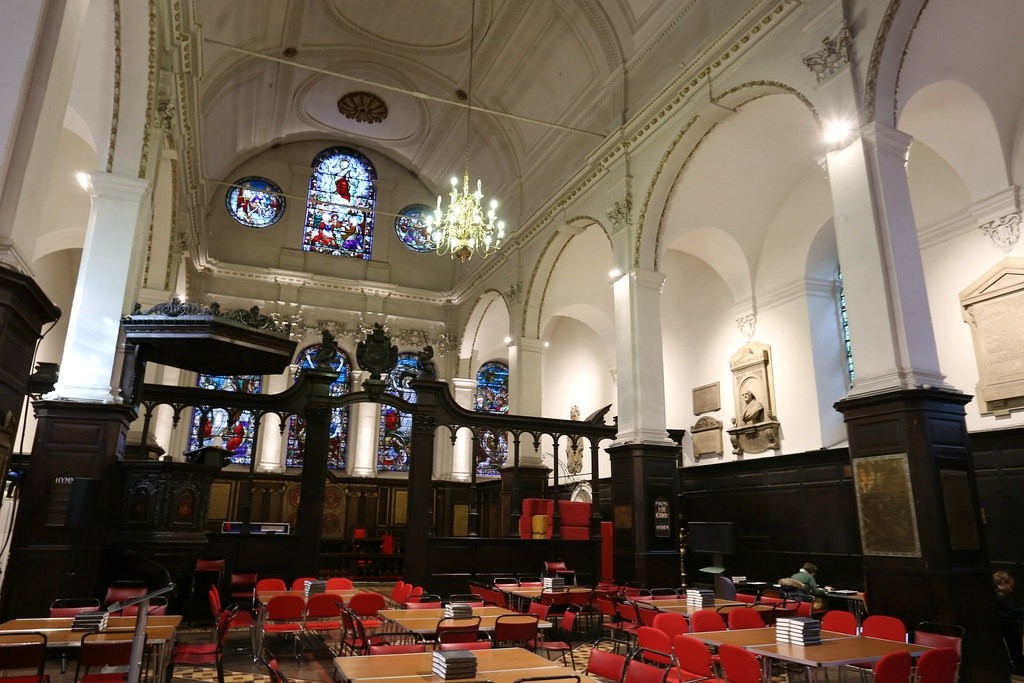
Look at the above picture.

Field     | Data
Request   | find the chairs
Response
[0,528,1024,683]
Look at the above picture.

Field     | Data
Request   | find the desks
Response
[332,645,603,679]
[626,596,789,608]
[512,589,592,612]
[258,594,355,605]
[659,602,789,617]
[681,625,939,649]
[496,585,593,592]
[376,604,554,620]
[815,588,867,628]
[0,625,176,683]
[254,588,366,597]
[744,636,936,683]
[394,613,552,645]
[351,666,602,683]
[0,614,183,631]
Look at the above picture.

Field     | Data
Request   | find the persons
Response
[741,389,764,424]
[992,570,1024,617]
[790,562,833,610]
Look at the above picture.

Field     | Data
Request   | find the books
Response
[444,604,474,620]
[71,611,110,632]
[432,651,478,680]
[776,617,822,647]
[686,589,715,608]
[542,577,565,592]
[305,580,327,598]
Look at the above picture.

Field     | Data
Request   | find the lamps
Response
[415,0,506,264]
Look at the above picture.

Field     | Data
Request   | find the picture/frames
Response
[849,452,923,561]
